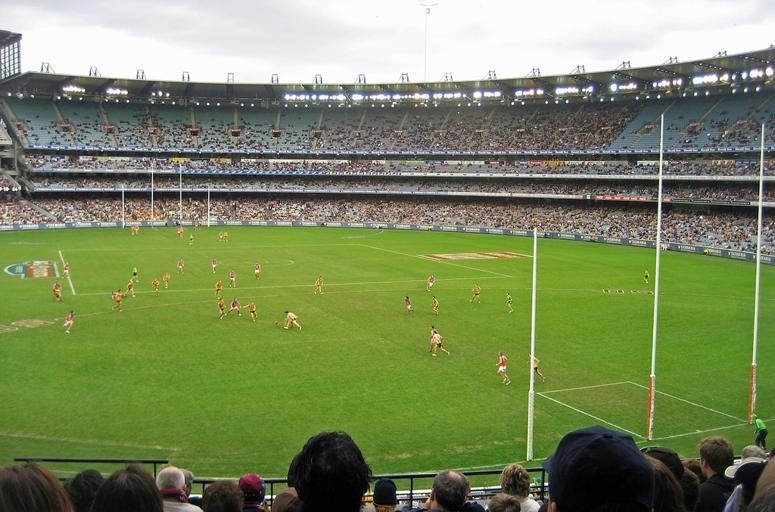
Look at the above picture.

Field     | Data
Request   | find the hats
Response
[236,473,266,503]
[725,456,769,480]
[372,479,398,506]
[640,446,684,479]
[541,425,654,508]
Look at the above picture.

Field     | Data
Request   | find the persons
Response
[643,269,650,284]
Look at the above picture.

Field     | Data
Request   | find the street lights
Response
[418,2,440,82]
